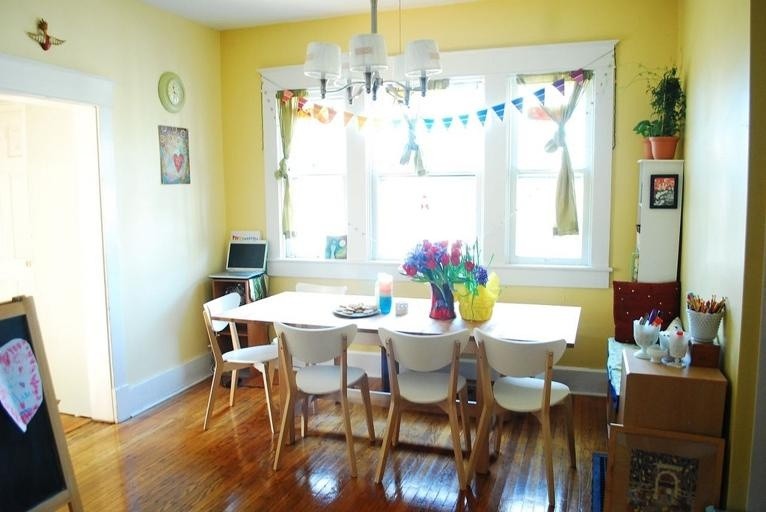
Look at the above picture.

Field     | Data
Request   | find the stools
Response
[606,331,690,402]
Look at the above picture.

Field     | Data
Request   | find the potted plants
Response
[632,64,687,160]
[647,123,681,160]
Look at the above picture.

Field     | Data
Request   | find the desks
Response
[210,290,586,476]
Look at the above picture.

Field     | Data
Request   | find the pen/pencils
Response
[639,309,667,326]
[687,292,727,314]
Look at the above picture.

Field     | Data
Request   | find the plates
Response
[333,303,379,318]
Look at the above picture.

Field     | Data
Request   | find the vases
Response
[427,281,457,323]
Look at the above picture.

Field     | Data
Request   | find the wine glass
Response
[633,321,689,368]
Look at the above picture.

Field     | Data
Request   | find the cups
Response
[396,302,408,316]
[687,309,723,344]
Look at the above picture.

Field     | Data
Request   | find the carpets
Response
[591,448,609,510]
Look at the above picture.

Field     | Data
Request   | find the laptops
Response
[208,240,268,280]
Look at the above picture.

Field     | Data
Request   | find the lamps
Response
[301,1,446,108]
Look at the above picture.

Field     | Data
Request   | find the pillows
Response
[611,277,685,344]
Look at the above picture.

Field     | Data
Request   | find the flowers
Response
[394,232,497,302]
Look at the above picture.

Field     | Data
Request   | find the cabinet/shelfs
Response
[209,275,268,379]
[629,157,686,283]
[610,353,731,435]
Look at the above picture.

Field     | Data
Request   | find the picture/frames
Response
[648,172,679,211]
[603,423,728,512]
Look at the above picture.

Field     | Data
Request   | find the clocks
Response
[155,69,190,115]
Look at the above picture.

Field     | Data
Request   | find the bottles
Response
[376,274,393,314]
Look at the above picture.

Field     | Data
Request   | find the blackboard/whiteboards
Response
[0,294,77,512]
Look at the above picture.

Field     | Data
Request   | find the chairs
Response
[271,317,377,478]
[464,326,583,511]
[267,282,352,411]
[200,292,287,439]
[371,324,476,491]
